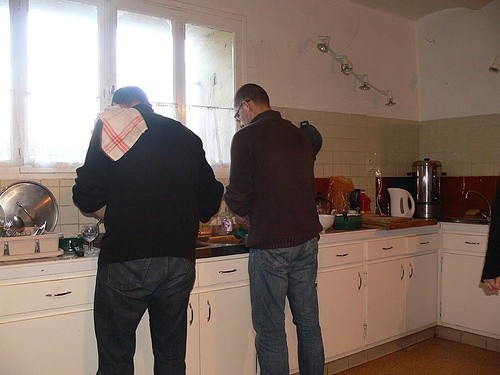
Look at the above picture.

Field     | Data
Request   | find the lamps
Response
[488,56,500,74]
[317,35,396,106]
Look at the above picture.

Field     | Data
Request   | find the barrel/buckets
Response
[411,157,445,204]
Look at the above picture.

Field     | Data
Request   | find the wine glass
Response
[81,223,99,255]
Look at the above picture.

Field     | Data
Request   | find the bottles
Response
[316,192,332,214]
[198,206,238,238]
[346,188,371,214]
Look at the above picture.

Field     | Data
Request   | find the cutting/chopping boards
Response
[360,213,437,230]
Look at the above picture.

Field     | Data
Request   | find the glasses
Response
[234,99,251,123]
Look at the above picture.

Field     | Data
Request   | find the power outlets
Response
[366,157,381,173]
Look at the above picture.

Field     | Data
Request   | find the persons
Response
[72,87,224,375]
[480,175,500,290]
[225,83,325,375]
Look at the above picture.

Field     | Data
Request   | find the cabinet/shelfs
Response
[0,226,500,375]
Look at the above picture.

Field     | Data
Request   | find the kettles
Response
[387,188,415,218]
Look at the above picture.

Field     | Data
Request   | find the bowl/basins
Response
[334,214,361,230]
[319,214,335,233]
